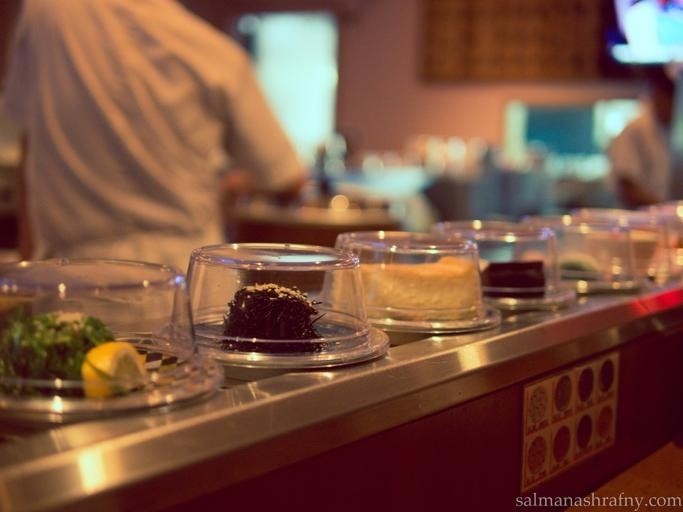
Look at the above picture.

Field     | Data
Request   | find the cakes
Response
[481,261,544,298]
[222,286,321,356]
[333,259,482,323]
[590,228,658,263]
[559,256,605,282]
[3,311,115,396]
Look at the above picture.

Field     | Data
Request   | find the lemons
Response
[81,340,150,400]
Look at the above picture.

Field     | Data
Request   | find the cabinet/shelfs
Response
[0,274,683,512]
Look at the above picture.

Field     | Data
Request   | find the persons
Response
[612,76,675,209]
[0,1,305,291]
